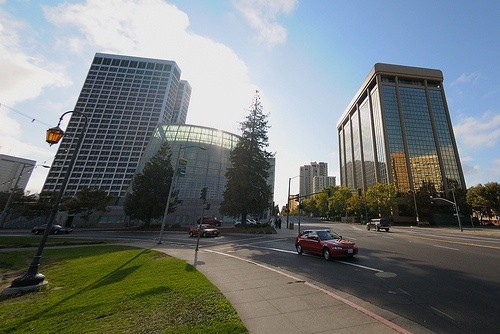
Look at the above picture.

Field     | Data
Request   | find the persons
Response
[275,219,281,229]
[270,218,272,224]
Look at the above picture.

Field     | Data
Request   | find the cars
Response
[197,216,222,226]
[234,218,261,226]
[31,224,72,235]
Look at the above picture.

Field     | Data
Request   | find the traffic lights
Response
[200,186,207,199]
[295,193,299,202]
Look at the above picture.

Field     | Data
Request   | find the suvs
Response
[366,218,391,232]
[295,228,358,260]
[189,224,219,238]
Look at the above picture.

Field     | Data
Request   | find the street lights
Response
[286,174,304,228]
[157,145,208,245]
[9,110,88,285]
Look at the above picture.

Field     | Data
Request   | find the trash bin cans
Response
[290,222,294,229]
[367,224,370,230]
